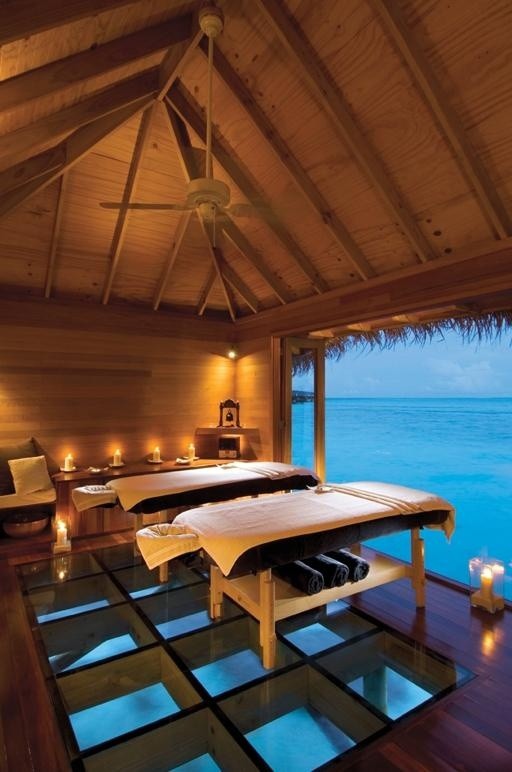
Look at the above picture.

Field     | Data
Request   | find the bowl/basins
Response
[13,562,51,578]
[0,513,49,537]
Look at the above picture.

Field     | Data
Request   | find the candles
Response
[153,446,160,461]
[480,567,494,599]
[57,520,67,546]
[114,449,121,466]
[188,443,195,459]
[65,453,73,470]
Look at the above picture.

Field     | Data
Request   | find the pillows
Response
[8,455,54,496]
[0,436,39,496]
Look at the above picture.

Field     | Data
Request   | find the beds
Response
[136,481,450,648]
[72,461,317,584]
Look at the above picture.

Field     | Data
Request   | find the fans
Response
[100,6,284,221]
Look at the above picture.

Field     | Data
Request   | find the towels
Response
[137,524,203,570]
[305,553,349,587]
[325,547,369,582]
[272,560,324,595]
[73,485,117,512]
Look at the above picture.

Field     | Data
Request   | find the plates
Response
[59,456,200,474]
